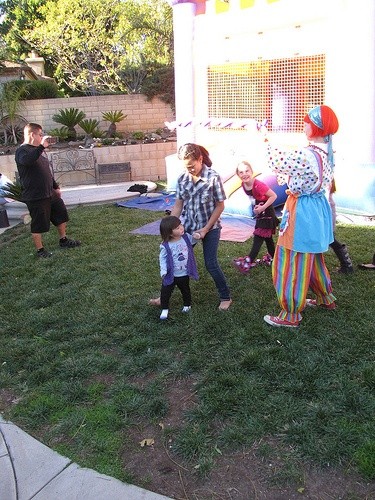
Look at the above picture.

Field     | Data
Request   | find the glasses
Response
[29,132,43,137]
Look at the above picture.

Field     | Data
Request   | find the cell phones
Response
[47,136,58,143]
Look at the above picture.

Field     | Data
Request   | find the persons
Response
[159,216,201,319]
[357,251,375,270]
[329,179,354,275]
[147,144,232,311]
[235,161,281,267]
[15,123,81,258]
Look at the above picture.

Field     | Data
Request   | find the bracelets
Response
[205,225,210,232]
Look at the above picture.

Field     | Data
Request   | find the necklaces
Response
[260,106,339,327]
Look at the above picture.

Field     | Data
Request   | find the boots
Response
[335,243,353,275]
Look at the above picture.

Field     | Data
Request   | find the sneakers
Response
[263,315,298,327]
[306,299,336,310]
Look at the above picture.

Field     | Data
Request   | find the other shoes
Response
[149,297,161,306]
[37,250,53,258]
[159,309,169,319]
[182,306,192,312]
[219,298,232,310]
[234,256,251,275]
[358,263,375,270]
[59,237,81,247]
[256,252,272,267]
